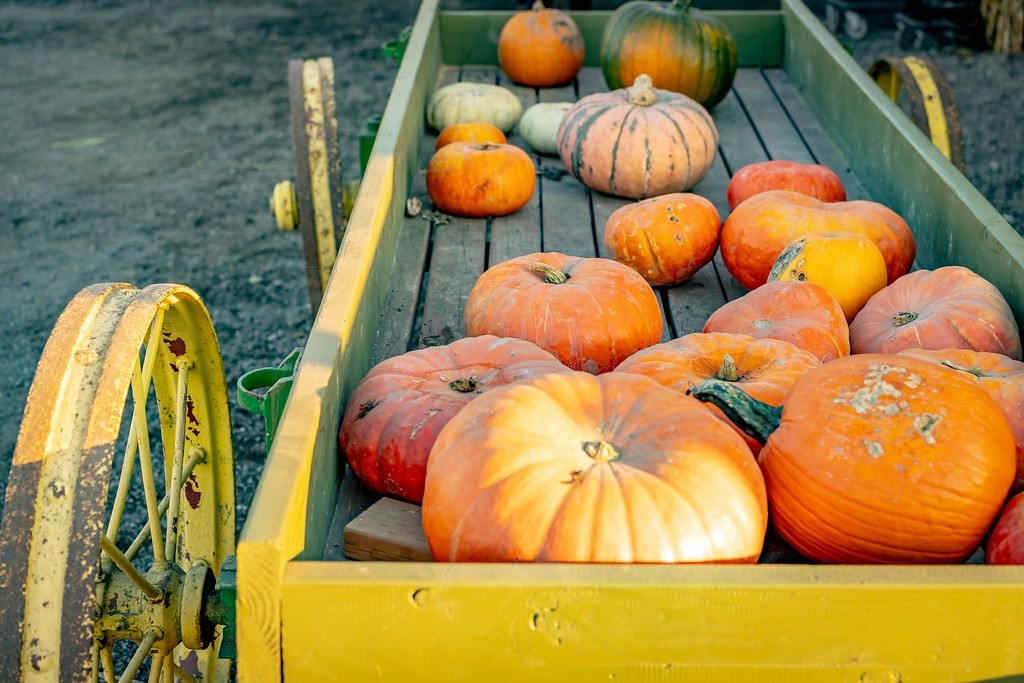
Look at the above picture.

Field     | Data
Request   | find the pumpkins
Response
[335,0,1024,566]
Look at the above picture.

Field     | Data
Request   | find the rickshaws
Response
[0,0,1024,682]
